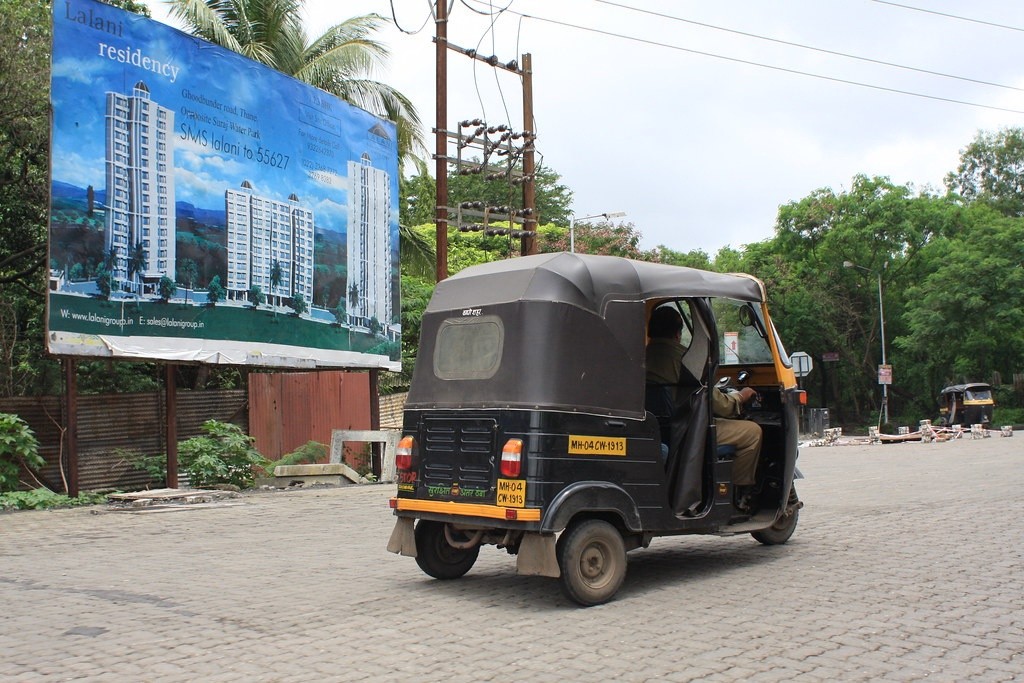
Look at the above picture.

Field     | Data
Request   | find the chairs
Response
[645,372,736,457]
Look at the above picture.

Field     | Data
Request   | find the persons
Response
[646,307,762,505]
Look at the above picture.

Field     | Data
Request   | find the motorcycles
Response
[382,248,810,608]
[936,383,995,430]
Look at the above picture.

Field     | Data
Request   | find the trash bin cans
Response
[808,407,830,435]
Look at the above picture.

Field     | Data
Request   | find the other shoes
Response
[737,487,758,513]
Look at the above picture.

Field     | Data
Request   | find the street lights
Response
[842,261,891,422]
[569,211,629,255]
